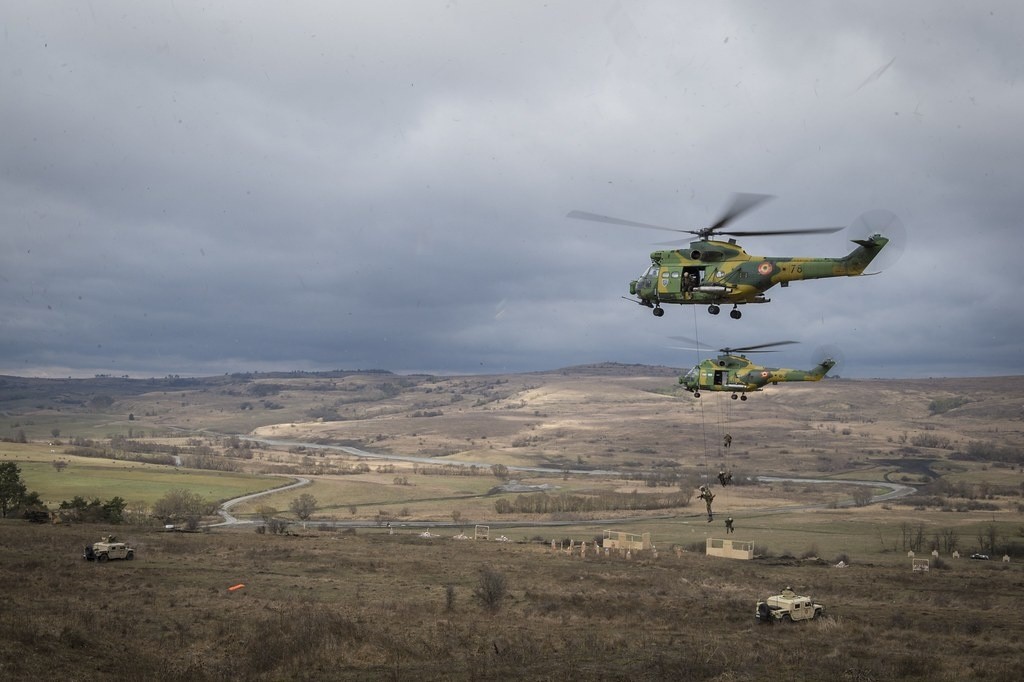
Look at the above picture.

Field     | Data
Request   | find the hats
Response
[729,518,732,521]
[684,272,689,277]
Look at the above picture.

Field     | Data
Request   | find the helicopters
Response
[570,189,892,320]
[668,331,839,402]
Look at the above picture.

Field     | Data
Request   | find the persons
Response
[724,433,732,448]
[724,473,732,486]
[717,471,727,487]
[699,486,713,523]
[725,517,734,534]
[681,272,696,300]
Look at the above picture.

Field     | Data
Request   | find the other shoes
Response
[680,296,685,300]
[689,295,692,299]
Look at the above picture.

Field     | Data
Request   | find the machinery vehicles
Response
[85,536,135,565]
[757,585,824,625]
